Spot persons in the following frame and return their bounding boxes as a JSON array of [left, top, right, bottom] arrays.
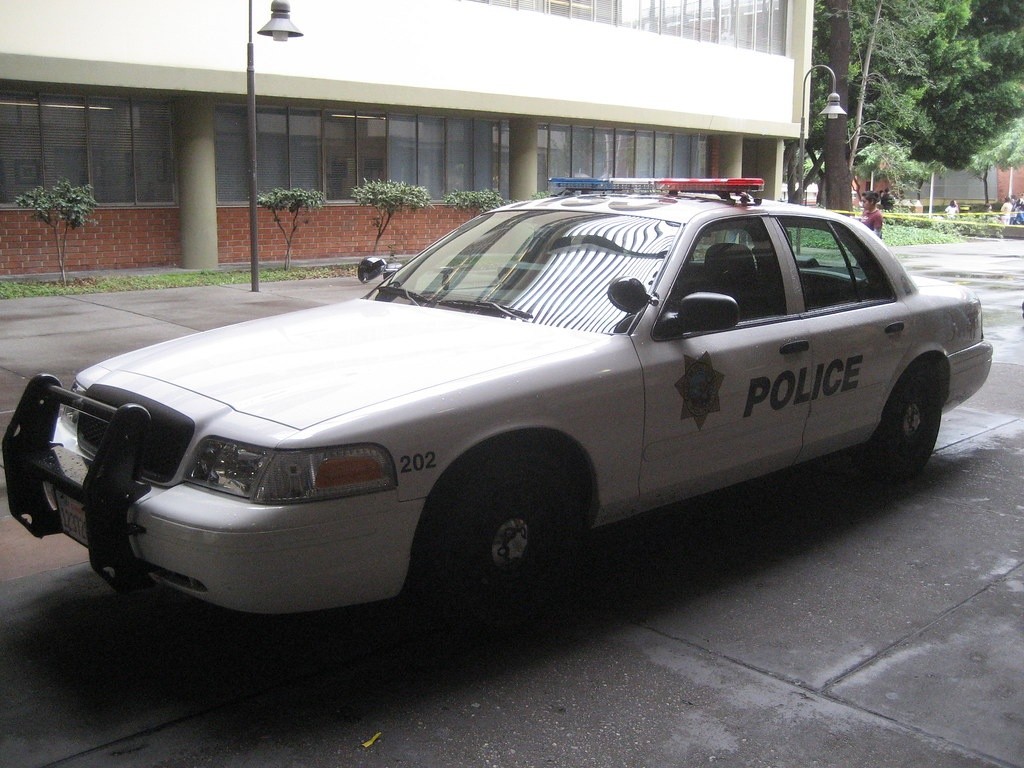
[[944, 200, 959, 218], [859, 191, 883, 240], [881, 187, 895, 209], [1001, 193, 1024, 225]]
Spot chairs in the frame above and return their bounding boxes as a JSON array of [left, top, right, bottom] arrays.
[[704, 242, 772, 323]]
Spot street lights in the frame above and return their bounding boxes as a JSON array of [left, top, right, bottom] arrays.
[[797, 66, 847, 257], [246, 0, 306, 290]]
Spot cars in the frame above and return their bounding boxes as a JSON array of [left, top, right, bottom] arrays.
[[3, 176, 993, 641]]
